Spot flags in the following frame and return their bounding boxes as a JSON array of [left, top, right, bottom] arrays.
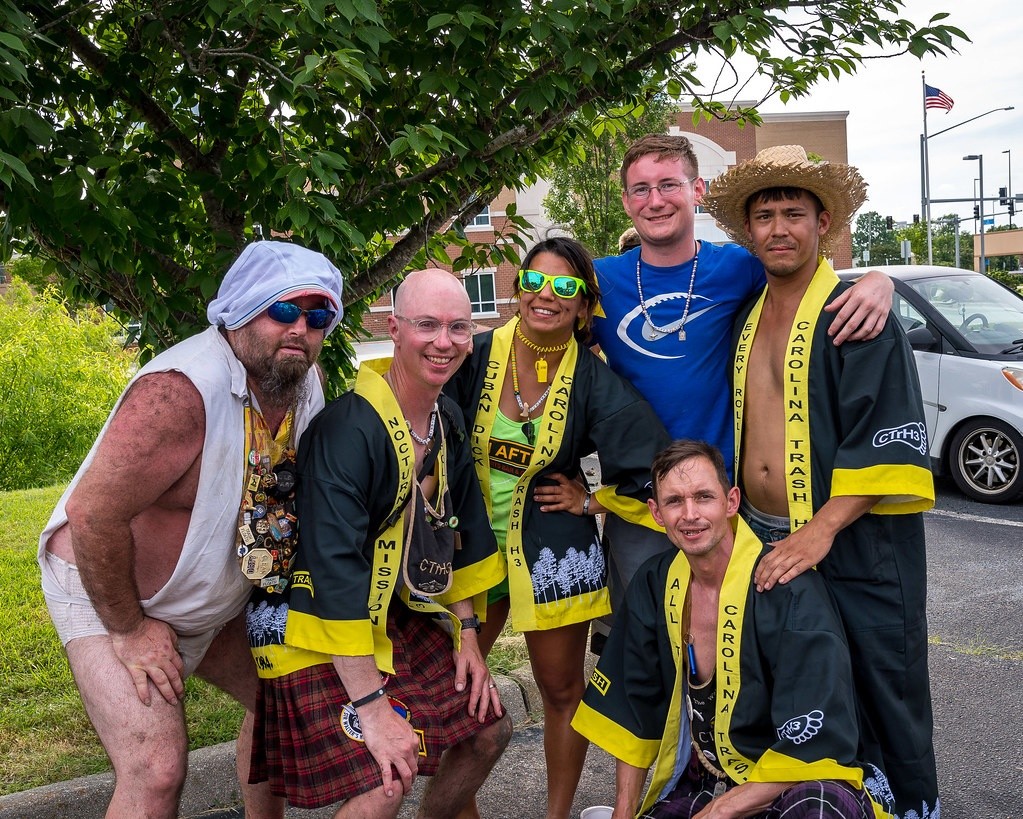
[[926, 84, 955, 114]]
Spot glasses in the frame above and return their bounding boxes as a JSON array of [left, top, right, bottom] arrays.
[[518, 268, 588, 298], [623, 176, 699, 201], [395, 314, 478, 344], [267, 302, 336, 330]]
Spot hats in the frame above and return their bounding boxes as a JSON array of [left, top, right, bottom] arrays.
[[701, 146, 869, 257], [281, 283, 337, 310]]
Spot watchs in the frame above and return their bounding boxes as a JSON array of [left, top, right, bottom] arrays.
[[460, 614, 481, 634]]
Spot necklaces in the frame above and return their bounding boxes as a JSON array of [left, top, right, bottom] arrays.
[[515, 321, 572, 351], [637, 239, 698, 341], [405, 411, 437, 444], [511, 340, 552, 412]]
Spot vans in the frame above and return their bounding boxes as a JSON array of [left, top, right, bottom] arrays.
[[833, 264, 1023, 505]]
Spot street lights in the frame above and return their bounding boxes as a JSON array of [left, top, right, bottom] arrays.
[[962, 154, 986, 275], [920, 106, 1014, 224], [1003, 150, 1012, 229]]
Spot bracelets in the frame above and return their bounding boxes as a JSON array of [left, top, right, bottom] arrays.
[[583, 493, 591, 514], [352, 687, 386, 708]]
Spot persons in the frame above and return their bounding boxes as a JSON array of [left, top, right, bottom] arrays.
[[701, 144, 941, 819], [441, 236, 673, 819], [245, 267, 513, 819], [37, 240, 343, 819], [569, 440, 864, 819], [468, 134, 895, 656]]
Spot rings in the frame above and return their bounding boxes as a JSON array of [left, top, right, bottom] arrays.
[[489, 684, 496, 689]]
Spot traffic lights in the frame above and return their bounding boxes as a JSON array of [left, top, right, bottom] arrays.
[[886, 217, 894, 230], [974, 204, 979, 220], [1007, 201, 1014, 216]]
